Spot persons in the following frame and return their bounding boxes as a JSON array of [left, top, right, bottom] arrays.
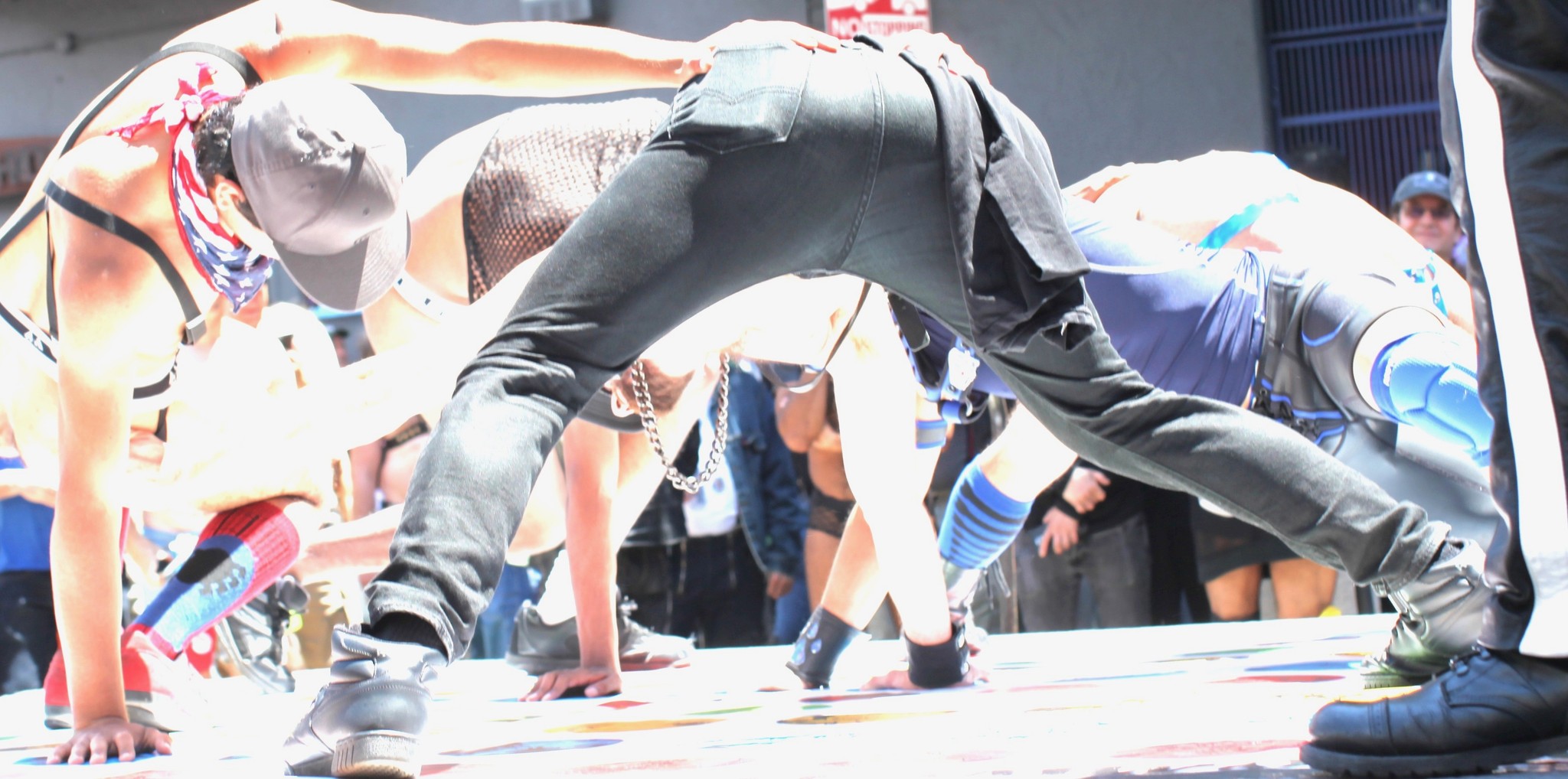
[[0, 0, 1568, 779]]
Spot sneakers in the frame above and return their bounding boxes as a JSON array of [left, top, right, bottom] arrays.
[[42, 648, 75, 728], [120, 625, 195, 732]]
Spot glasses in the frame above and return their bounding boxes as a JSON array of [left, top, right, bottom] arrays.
[[1406, 207, 1454, 219]]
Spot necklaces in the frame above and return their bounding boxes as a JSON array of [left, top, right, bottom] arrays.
[[631, 344, 730, 493]]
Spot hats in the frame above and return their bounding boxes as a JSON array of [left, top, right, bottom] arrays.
[[1391, 171, 1462, 217], [231, 74, 410, 312]]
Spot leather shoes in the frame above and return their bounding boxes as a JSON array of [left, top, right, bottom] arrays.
[[215, 574, 307, 696], [1343, 538, 1488, 694], [1299, 648, 1568, 778], [505, 596, 694, 674], [278, 626, 445, 777]]
